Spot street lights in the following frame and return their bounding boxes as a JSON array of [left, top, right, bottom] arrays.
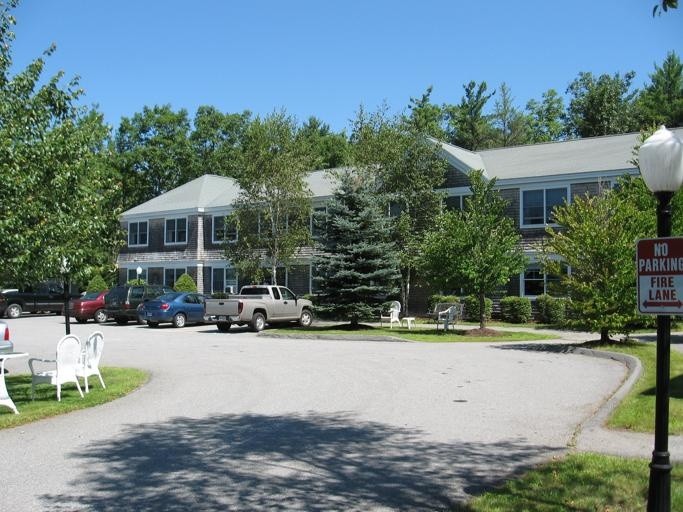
[[637, 126, 683, 512]]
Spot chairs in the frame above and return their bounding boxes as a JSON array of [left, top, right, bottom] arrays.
[[26, 330, 105, 402], [380, 299, 402, 329], [436, 306, 457, 331]]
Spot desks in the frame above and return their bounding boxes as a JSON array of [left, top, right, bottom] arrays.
[[401, 317, 416, 330], [0, 351, 29, 415]]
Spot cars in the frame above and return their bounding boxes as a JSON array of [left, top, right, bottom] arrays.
[[136, 290, 208, 328], [0, 320, 15, 361], [69, 288, 111, 325]]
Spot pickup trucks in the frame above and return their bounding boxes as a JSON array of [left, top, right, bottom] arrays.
[[0, 282, 82, 319], [204, 284, 315, 332]]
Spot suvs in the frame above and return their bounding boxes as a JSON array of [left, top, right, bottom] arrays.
[[104, 285, 173, 325]]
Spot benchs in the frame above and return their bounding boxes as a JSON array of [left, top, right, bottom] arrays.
[[427, 302, 464, 324]]
